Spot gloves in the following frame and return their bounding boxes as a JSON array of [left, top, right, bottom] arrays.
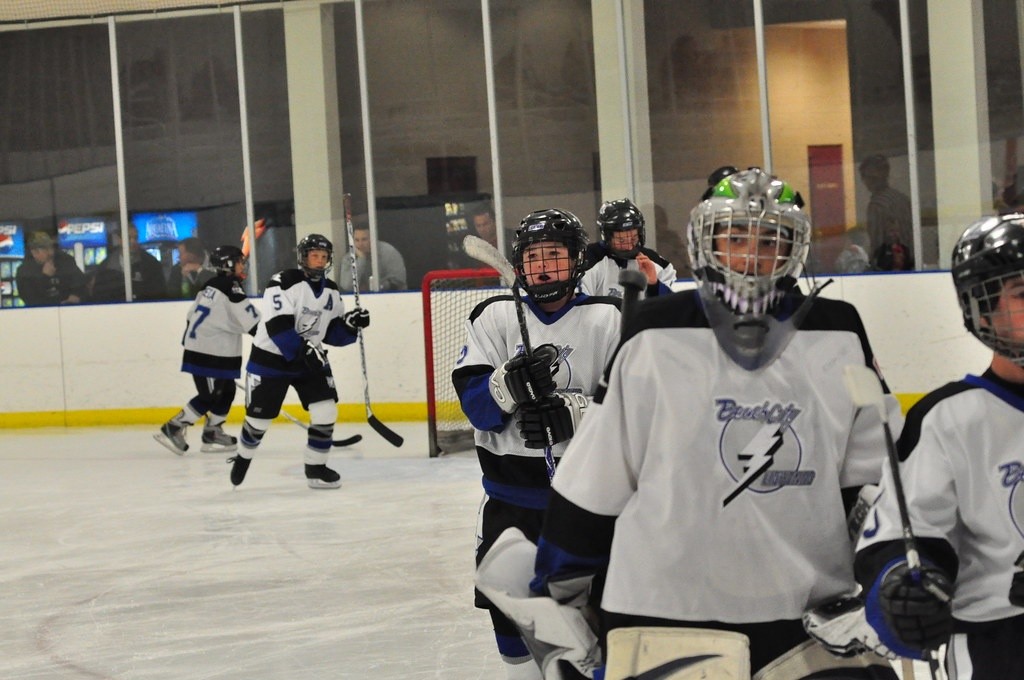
[[345, 308, 369, 328], [490, 353, 557, 415], [304, 340, 325, 374], [882, 564, 952, 650], [515, 393, 589, 449]]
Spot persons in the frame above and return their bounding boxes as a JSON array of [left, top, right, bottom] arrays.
[[91, 224, 165, 303], [164, 236, 216, 300], [16, 231, 89, 307], [528, 171, 902, 680], [339, 223, 406, 292], [450, 209, 625, 680], [226, 234, 371, 485], [852, 214, 1024, 680], [447, 207, 518, 290], [160, 245, 262, 451], [573, 199, 677, 303], [844, 152, 913, 276], [868, 220, 915, 271], [654, 204, 694, 278]]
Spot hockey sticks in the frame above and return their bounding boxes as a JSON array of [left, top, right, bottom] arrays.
[[343, 191, 405, 449], [232, 376, 363, 447], [845, 363, 951, 680], [462, 234, 558, 487]]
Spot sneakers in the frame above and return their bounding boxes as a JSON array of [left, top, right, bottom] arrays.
[[153, 409, 194, 456], [227, 454, 252, 489], [200, 413, 237, 452], [305, 463, 341, 488]]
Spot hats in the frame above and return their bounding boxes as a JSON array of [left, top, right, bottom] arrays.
[[27, 231, 58, 248]]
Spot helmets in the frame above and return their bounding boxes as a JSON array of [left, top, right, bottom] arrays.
[[296, 234, 335, 281], [596, 198, 646, 260], [951, 213, 1024, 369], [210, 245, 248, 280], [511, 208, 590, 303], [686, 168, 810, 315]]
